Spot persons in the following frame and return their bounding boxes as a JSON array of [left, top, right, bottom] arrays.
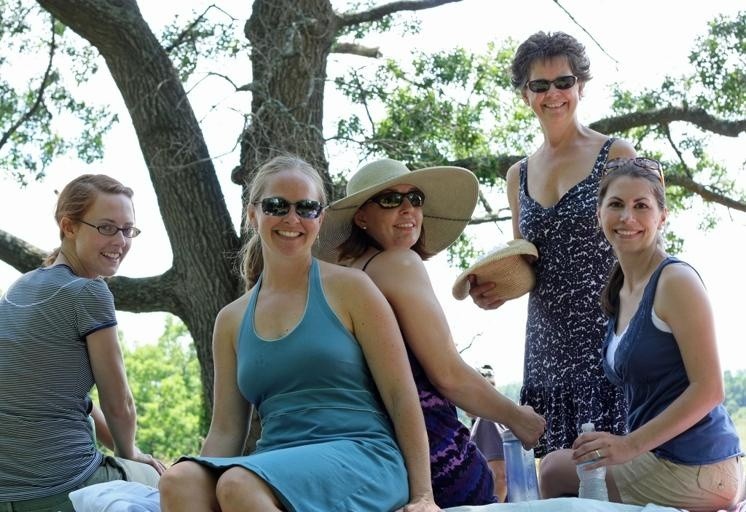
[[462, 363, 508, 504], [0, 172, 171, 512], [462, 30, 640, 499], [156, 153, 444, 510], [334, 182, 549, 508], [530, 152, 744, 511]]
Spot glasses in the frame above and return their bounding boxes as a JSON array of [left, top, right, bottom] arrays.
[[70, 217, 142, 239], [249, 197, 324, 221], [599, 156, 666, 199], [526, 73, 579, 94], [361, 187, 426, 209]]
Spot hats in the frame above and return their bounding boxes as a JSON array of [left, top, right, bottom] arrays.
[[451, 238, 541, 304], [310, 158, 479, 268]]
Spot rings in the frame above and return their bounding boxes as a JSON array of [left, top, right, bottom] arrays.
[[592, 448, 602, 458]]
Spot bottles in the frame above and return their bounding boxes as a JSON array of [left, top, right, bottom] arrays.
[[575, 423, 610, 498], [501, 428, 540, 502]]
